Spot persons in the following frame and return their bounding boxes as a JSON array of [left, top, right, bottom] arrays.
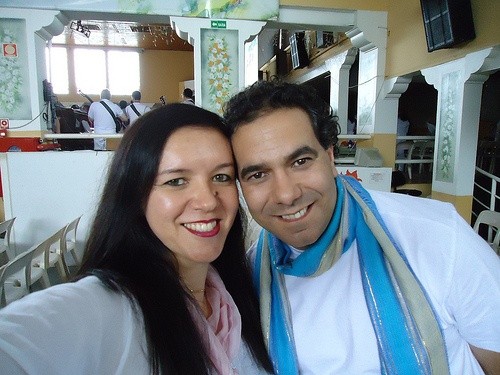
[[47, 84, 195, 151], [0, 105, 278, 375], [223, 77, 500, 375]]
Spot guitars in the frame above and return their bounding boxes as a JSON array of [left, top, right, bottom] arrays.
[[78, 90, 93, 104], [159, 95, 169, 105]]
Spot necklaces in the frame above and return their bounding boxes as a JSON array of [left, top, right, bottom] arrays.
[[183, 281, 207, 297]]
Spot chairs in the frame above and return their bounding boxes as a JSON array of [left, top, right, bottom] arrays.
[[472, 210, 500, 254], [397, 140, 433, 179], [0, 216, 82, 307]]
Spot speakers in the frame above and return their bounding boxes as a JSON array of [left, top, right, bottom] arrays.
[[419, 0, 476, 52], [289, 32, 309, 70]]
